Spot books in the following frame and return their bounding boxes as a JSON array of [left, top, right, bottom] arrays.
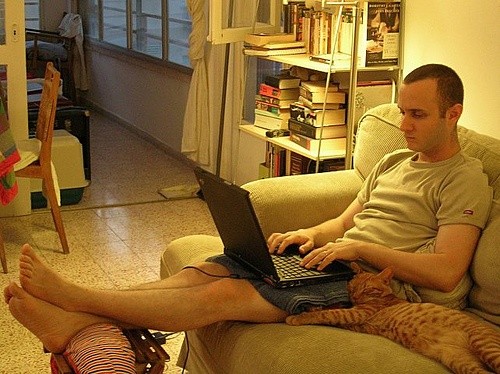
[[254, 76, 300, 130], [287, 79, 348, 150], [242, 1, 399, 65], [257, 142, 346, 180]]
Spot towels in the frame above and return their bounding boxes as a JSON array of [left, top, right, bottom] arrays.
[[59, 13, 88, 91]]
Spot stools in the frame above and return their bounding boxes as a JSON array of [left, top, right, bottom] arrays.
[[43, 325, 170, 374]]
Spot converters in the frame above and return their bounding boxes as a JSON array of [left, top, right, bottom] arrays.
[[152, 332, 166, 344]]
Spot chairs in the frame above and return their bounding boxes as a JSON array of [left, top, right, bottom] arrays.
[[0, 62, 69, 274], [25, 11, 73, 83]]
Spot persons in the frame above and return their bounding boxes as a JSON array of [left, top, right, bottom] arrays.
[[3, 64, 495, 355]]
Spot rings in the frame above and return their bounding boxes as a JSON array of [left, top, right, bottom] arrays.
[[323, 251, 328, 255]]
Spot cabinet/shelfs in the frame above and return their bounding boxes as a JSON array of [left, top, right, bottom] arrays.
[[233, 0, 405, 187]]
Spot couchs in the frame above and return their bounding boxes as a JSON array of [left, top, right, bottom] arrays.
[[160, 103, 500, 374]]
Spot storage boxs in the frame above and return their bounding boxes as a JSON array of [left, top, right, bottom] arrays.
[[28, 129, 89, 209]]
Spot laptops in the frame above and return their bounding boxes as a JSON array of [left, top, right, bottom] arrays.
[[194, 166, 354, 289]]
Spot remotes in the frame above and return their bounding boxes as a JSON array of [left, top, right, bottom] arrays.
[[266, 129, 290, 138]]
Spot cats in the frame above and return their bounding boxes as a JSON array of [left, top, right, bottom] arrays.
[[285, 261, 500, 374]]
